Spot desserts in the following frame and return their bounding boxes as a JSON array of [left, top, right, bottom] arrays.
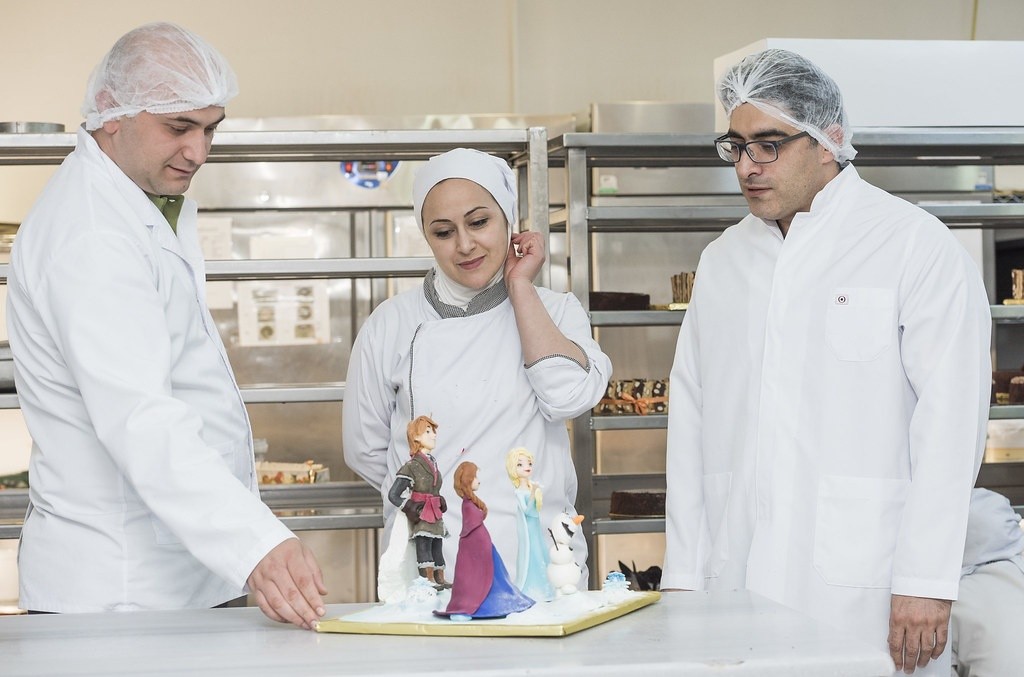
[[590, 291, 651, 311]]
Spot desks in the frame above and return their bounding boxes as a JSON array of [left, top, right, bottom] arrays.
[[0, 590, 899, 677]]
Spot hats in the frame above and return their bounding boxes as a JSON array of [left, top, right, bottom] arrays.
[[413, 148, 516, 237]]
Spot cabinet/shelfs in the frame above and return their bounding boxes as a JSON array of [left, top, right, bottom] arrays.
[[506, 133, 1024, 590], [0, 127, 549, 605]]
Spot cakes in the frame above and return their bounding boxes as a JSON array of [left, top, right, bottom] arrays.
[[610, 488, 667, 516], [592, 378, 670, 415], [670, 271, 696, 303]]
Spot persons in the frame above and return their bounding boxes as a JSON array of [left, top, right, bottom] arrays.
[[10, 25, 330, 636], [661, 52, 993, 677], [341, 149, 614, 605], [950, 488, 1021, 676], [389, 416, 549, 623]]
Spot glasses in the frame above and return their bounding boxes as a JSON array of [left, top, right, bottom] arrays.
[[714, 128, 808, 164]]
